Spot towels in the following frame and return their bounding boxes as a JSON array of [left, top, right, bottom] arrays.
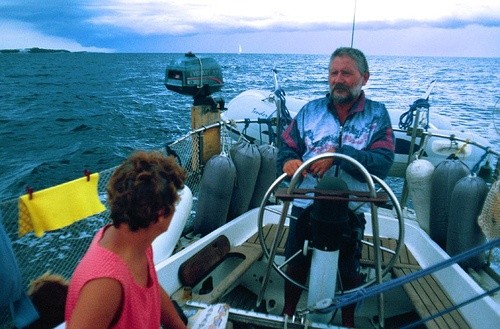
[[18, 172, 108, 238]]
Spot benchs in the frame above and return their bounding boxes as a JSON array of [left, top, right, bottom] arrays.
[[393, 268, 471, 329], [242, 223, 421, 269]]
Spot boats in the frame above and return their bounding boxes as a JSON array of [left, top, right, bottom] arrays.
[[219, 90, 491, 163]]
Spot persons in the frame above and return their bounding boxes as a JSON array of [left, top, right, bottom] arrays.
[[277, 47, 396, 329], [65, 152, 186, 329]]
